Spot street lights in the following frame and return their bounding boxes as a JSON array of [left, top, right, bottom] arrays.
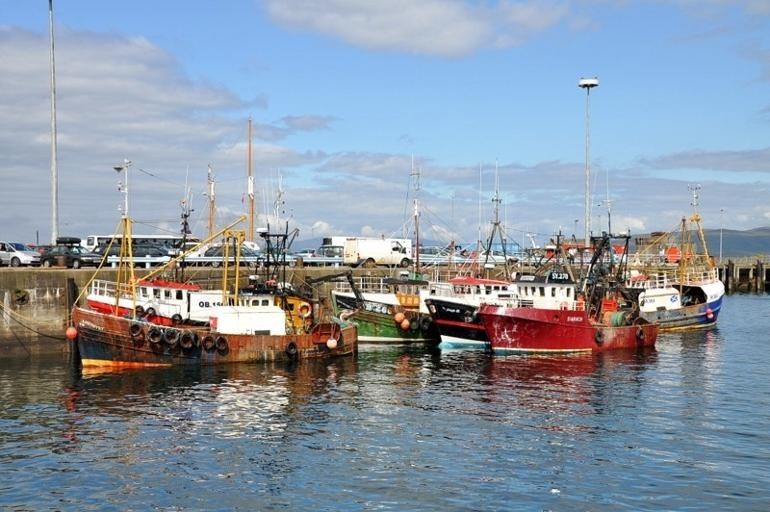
[[578, 76, 601, 251]]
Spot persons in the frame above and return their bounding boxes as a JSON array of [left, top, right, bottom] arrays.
[[634, 249, 642, 267], [567, 245, 578, 264]]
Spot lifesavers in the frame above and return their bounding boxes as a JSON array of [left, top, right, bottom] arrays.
[[297, 302, 312, 318]]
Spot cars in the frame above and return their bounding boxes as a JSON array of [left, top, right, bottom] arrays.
[[0, 241, 42, 267], [41, 237, 106, 270]]
